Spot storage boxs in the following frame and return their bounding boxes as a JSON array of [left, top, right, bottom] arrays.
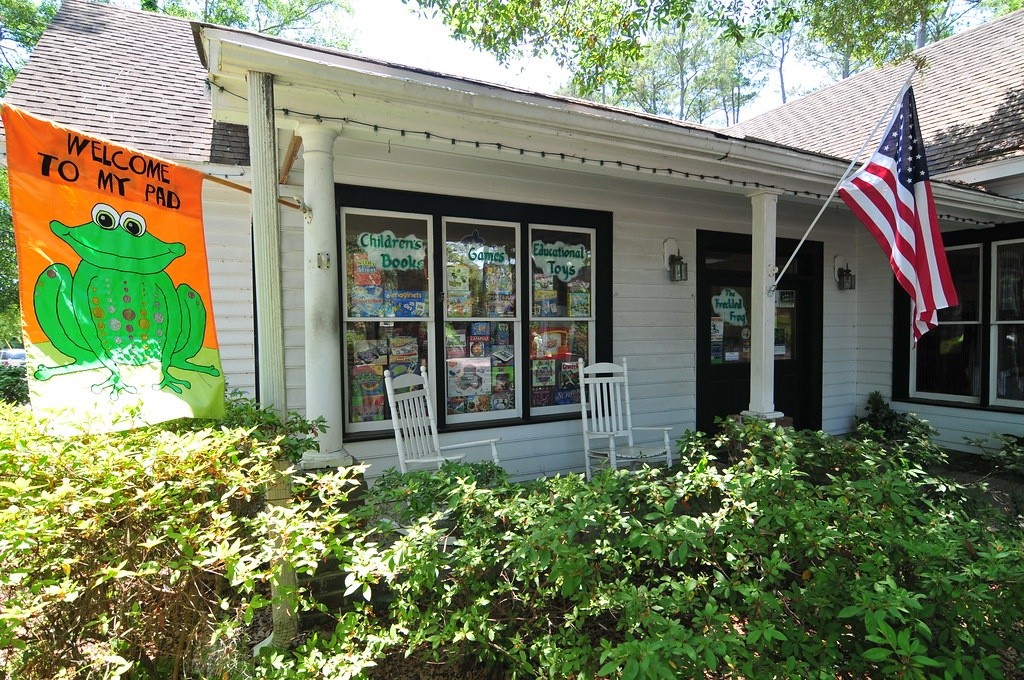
[[347, 254, 590, 423]]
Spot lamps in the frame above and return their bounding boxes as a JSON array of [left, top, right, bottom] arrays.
[[664, 236, 689, 284], [834, 253, 856, 291]]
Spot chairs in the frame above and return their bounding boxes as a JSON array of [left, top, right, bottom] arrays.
[[578, 357, 674, 481], [384, 365, 502, 472]]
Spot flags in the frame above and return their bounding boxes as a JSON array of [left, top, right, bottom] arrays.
[[837, 79, 959, 351]]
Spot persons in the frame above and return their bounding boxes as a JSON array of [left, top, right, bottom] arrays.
[[916, 291, 977, 397]]
